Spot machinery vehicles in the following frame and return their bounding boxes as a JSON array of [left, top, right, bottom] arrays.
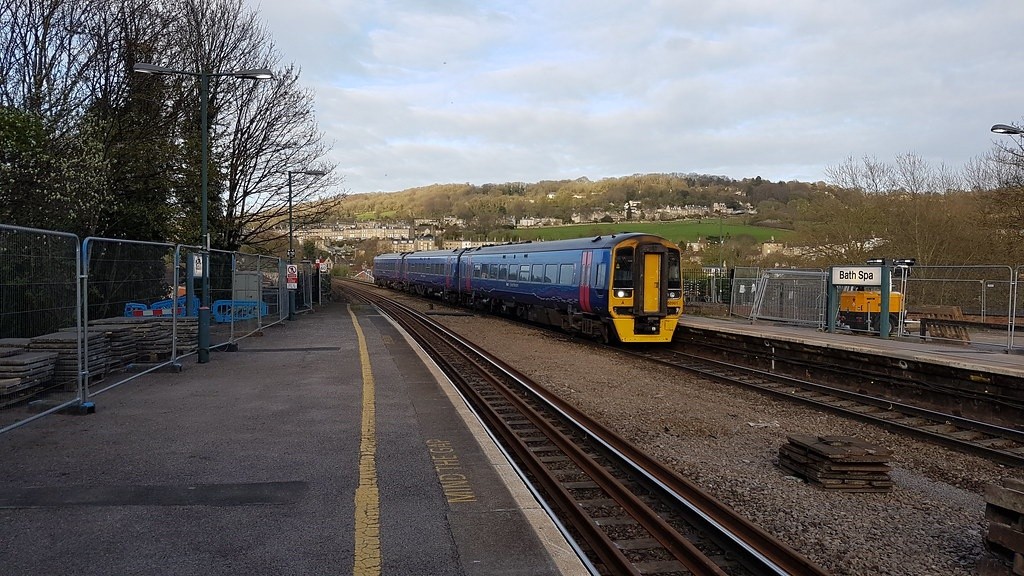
[[840, 260, 921, 337]]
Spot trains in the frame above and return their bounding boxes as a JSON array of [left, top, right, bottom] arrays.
[[372, 231, 684, 346]]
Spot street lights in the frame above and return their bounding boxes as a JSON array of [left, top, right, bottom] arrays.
[[714, 209, 723, 303], [289, 171, 325, 322], [132, 63, 273, 363]]
